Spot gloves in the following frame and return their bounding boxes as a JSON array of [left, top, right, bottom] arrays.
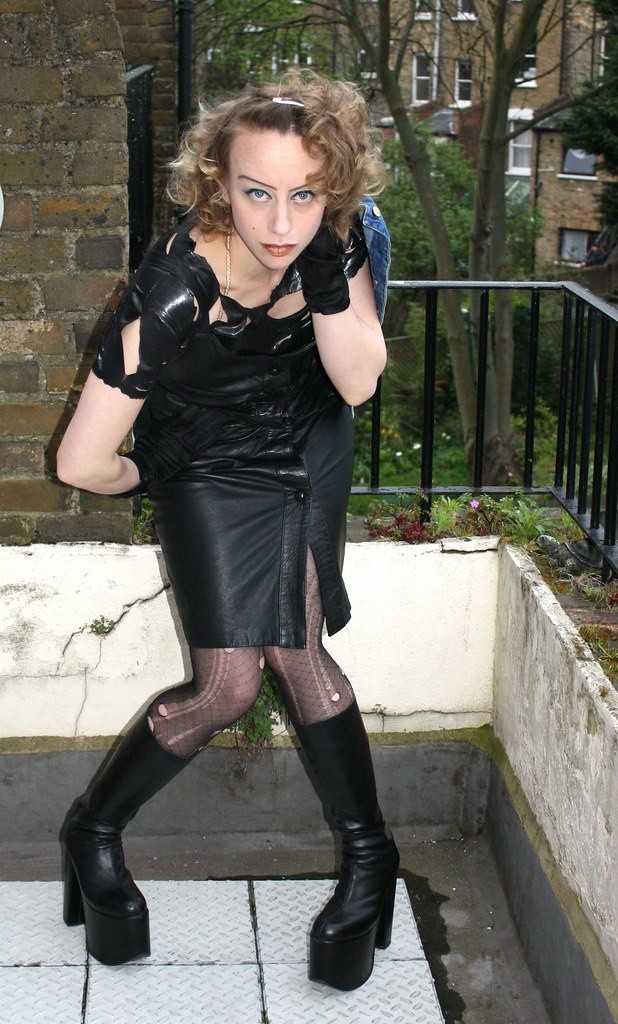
[[295, 218, 352, 316], [110, 404, 225, 499]]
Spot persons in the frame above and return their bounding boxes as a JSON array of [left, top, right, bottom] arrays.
[[57, 66, 401, 991]]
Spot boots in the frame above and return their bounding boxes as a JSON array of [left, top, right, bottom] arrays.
[[286, 671, 397, 991], [59, 707, 203, 966]]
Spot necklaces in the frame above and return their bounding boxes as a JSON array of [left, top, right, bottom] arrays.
[[218, 224, 231, 320]]
